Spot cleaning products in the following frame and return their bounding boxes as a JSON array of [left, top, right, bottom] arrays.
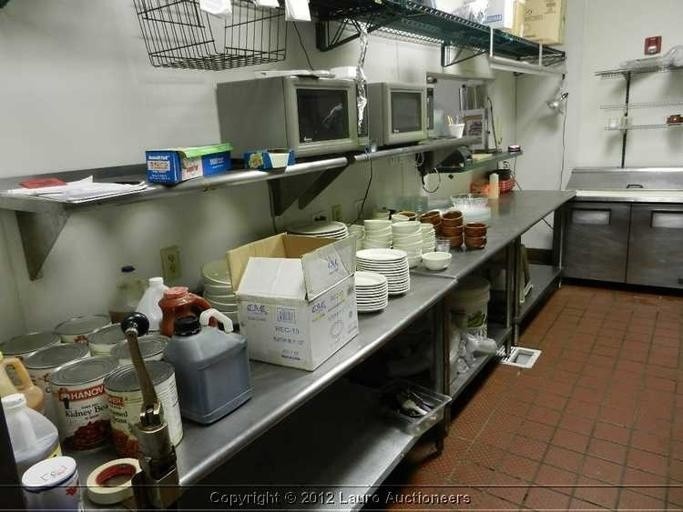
[[490, 171, 499, 199]]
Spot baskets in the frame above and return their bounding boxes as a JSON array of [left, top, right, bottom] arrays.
[[132, 1, 288, 72]]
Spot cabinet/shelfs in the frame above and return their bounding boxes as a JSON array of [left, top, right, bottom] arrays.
[[552, 194, 683, 288], [0, 0, 564, 282], [593, 62, 683, 135]]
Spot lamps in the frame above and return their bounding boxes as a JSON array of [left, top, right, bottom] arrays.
[[542, 86, 569, 115]]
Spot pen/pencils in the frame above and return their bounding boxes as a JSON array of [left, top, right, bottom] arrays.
[[447, 114, 458, 125]]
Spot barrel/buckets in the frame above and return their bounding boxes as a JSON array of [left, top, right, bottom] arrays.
[[2, 392, 62, 477], [136, 277, 166, 330], [165, 308, 253, 425], [0, 352, 45, 415], [449, 278, 491, 342], [161, 289, 219, 333]]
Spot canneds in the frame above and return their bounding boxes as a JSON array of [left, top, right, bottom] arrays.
[[0, 314, 185, 460]]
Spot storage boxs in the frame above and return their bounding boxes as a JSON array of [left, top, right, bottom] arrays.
[[225, 230, 361, 373]]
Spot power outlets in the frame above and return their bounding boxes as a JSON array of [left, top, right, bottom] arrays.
[[160, 246, 181, 281]]
